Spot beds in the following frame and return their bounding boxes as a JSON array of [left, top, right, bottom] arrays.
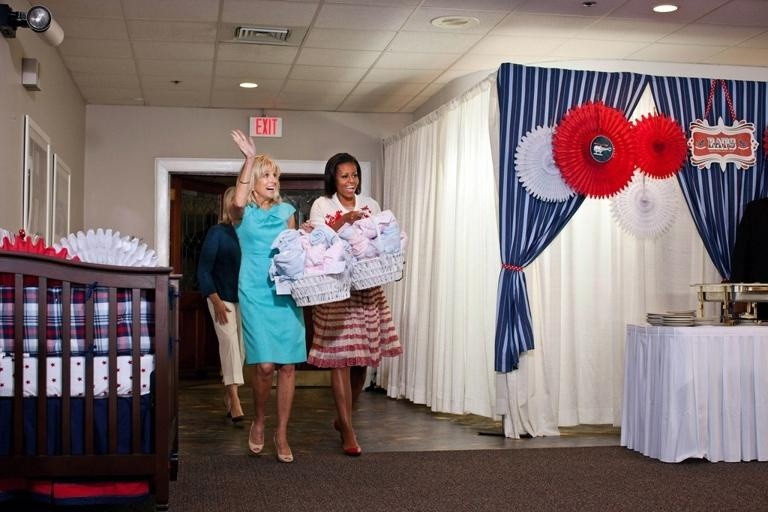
[[0, 222, 190, 511]]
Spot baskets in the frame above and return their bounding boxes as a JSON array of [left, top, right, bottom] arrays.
[[349, 249, 403, 292], [288, 266, 351, 307]]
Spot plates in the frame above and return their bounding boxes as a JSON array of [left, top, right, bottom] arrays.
[[647, 311, 697, 327]]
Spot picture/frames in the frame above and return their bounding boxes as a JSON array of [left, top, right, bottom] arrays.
[[49, 152, 74, 250], [18, 114, 54, 248]]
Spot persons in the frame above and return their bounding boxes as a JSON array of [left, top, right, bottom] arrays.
[[196, 185, 248, 425], [227, 129, 309, 464], [309, 153, 406, 458], [729, 194, 768, 321]]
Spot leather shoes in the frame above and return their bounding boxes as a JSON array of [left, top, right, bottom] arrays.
[[335, 417, 362, 456], [223, 400, 245, 422]]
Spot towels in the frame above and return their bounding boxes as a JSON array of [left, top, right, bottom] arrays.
[[269, 208, 409, 295]]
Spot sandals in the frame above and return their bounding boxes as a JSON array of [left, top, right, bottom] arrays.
[[273, 432, 294, 463], [249, 421, 264, 454]]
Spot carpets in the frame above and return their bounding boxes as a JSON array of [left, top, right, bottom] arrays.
[[161, 436, 766, 511]]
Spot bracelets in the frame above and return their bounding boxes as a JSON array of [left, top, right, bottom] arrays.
[[238, 177, 251, 185]]
